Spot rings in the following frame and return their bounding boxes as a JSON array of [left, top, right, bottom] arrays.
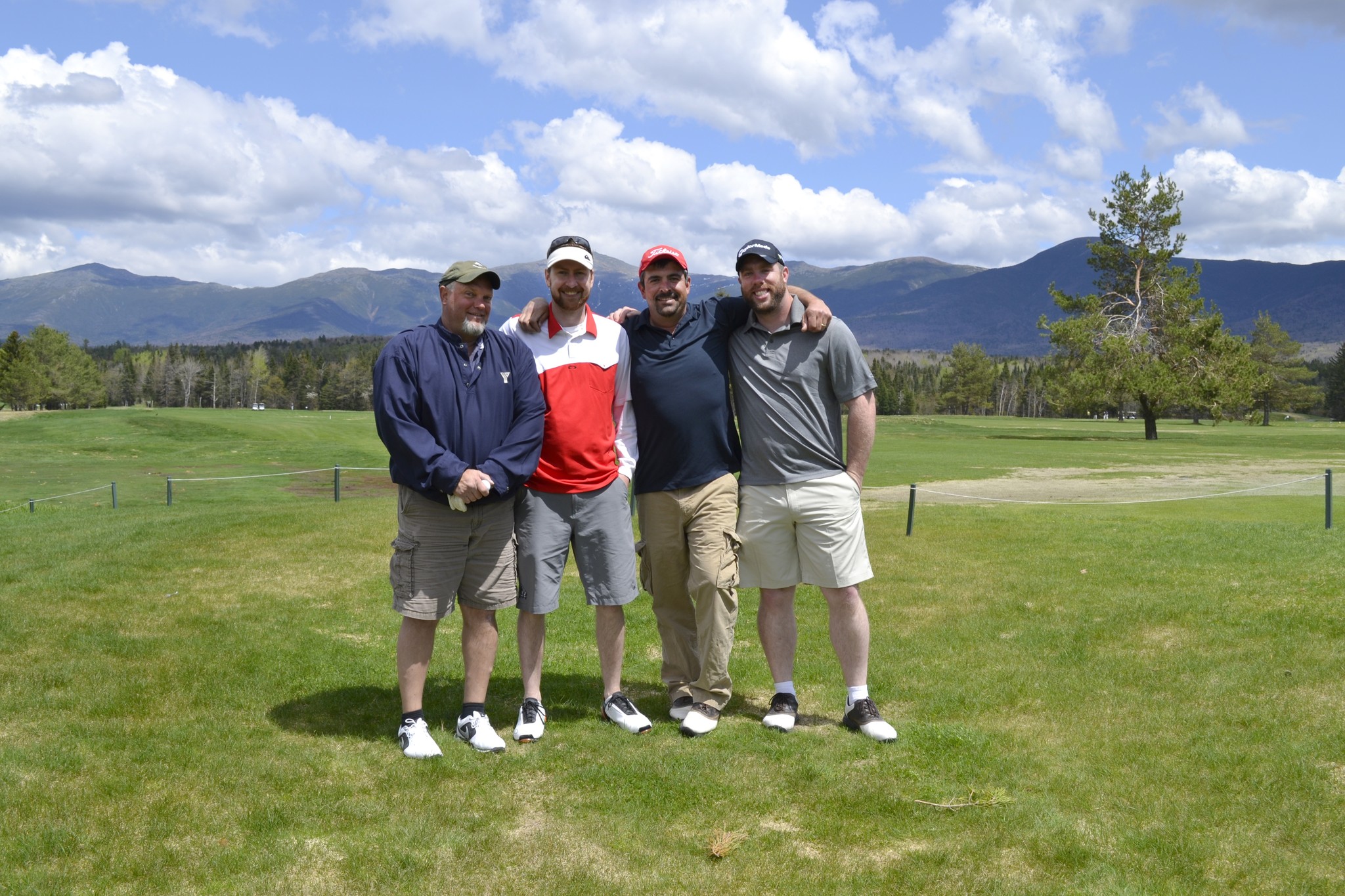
[[822, 323, 827, 327]]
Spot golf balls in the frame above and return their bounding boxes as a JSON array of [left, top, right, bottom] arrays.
[[482, 480, 491, 491]]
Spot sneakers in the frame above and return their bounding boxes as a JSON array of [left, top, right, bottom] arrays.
[[454, 711, 506, 754], [669, 695, 694, 722], [762, 693, 799, 733], [842, 695, 898, 744], [679, 702, 720, 738], [513, 697, 546, 744], [602, 691, 653, 736], [398, 717, 444, 760]]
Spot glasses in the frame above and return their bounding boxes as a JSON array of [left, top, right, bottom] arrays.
[[548, 236, 591, 256]]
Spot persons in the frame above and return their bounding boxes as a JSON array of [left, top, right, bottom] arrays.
[[606, 239, 899, 744], [1103, 412, 1106, 419], [1093, 412, 1097, 419], [519, 244, 834, 738], [290, 403, 294, 410], [1106, 410, 1108, 419], [370, 260, 548, 763], [496, 236, 655, 745]]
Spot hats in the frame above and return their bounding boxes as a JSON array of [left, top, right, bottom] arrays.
[[736, 239, 785, 276], [639, 245, 688, 279], [439, 260, 501, 290], [547, 246, 594, 270]]
